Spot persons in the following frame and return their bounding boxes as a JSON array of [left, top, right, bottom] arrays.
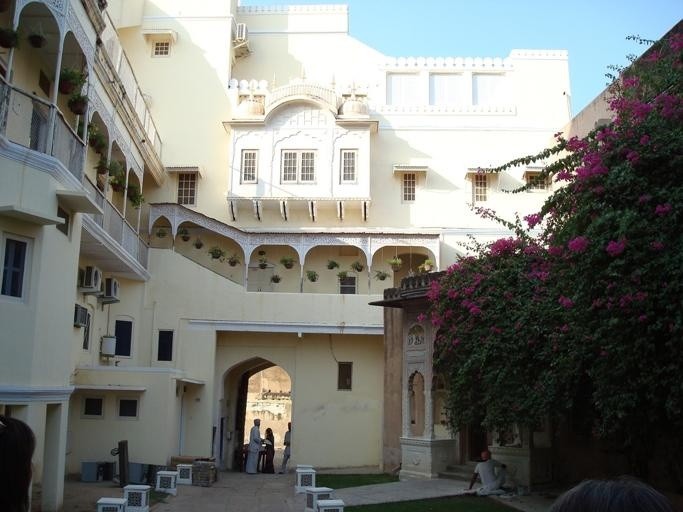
[[277, 421, 291, 474], [264, 428, 275, 474], [245, 418, 265, 474], [461, 449, 517, 498], [548, 469, 672, 512]]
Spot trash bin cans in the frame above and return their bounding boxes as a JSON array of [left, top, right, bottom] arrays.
[[193, 462, 215, 487]]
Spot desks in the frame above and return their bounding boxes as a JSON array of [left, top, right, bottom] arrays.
[[237, 444, 266, 474]]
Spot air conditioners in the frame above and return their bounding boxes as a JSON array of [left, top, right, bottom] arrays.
[[84, 264, 104, 298], [102, 277, 120, 304]]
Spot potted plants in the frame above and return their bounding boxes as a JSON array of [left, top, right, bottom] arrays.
[[13, 23, 146, 213], [152, 222, 432, 283]]
